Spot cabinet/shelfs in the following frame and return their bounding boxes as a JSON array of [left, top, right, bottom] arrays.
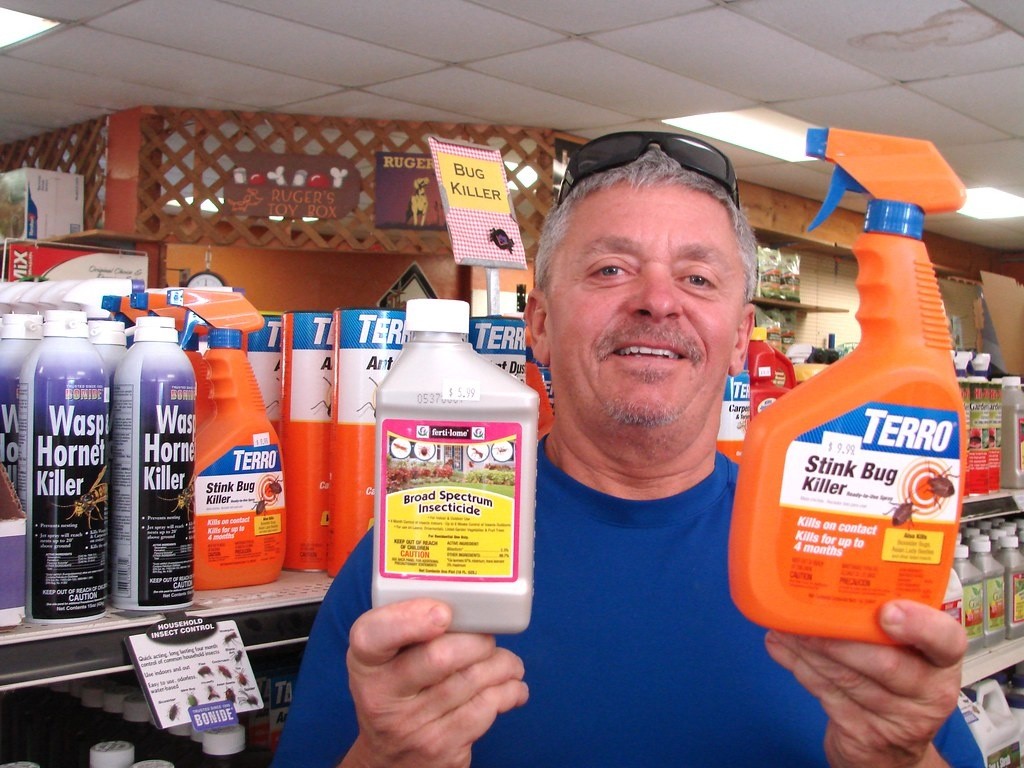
[[0, 488, 1024, 768]]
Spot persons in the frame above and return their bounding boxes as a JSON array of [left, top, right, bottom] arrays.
[[269, 132, 984, 768]]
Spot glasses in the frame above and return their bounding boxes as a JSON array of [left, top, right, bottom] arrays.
[[556, 130, 739, 210]]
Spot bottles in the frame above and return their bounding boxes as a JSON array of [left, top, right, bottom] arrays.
[[111, 316, 196, 610], [0, 312, 45, 631], [371, 299, 541, 635], [0, 674, 253, 768], [936, 517, 1024, 656], [747, 327, 797, 423], [18, 311, 110, 624], [1000, 376, 1024, 489], [957, 664, 1024, 768], [87, 319, 129, 376]]
